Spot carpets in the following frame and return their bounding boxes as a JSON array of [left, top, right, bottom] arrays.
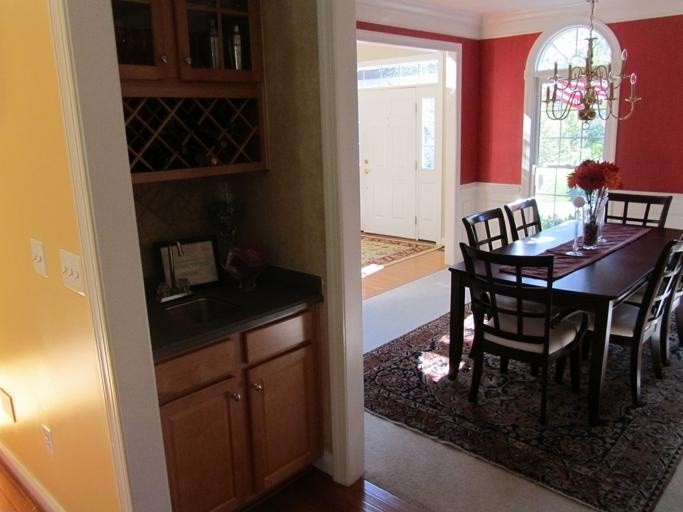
[[361, 233, 443, 280], [362, 299, 683, 512]]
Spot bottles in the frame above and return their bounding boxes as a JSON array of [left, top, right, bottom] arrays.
[[203, 19, 242, 71]]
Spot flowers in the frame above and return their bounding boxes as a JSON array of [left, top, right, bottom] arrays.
[[567, 160, 621, 226]]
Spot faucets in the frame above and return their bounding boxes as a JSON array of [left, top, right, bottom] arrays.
[[168, 240, 188, 291]]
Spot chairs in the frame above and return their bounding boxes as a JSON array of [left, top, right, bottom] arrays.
[[458, 242, 591, 425], [462, 197, 542, 251], [604, 192, 673, 228], [588, 240, 681, 407]]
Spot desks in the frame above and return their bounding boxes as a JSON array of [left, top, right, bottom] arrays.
[[448, 221, 683, 427]]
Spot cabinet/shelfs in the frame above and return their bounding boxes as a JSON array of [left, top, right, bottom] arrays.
[[113, 0, 272, 183], [154, 309, 323, 512]]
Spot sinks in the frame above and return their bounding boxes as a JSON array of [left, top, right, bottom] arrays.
[[162, 296, 241, 323]]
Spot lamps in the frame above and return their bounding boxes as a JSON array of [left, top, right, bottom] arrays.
[[541, 0, 641, 130]]
